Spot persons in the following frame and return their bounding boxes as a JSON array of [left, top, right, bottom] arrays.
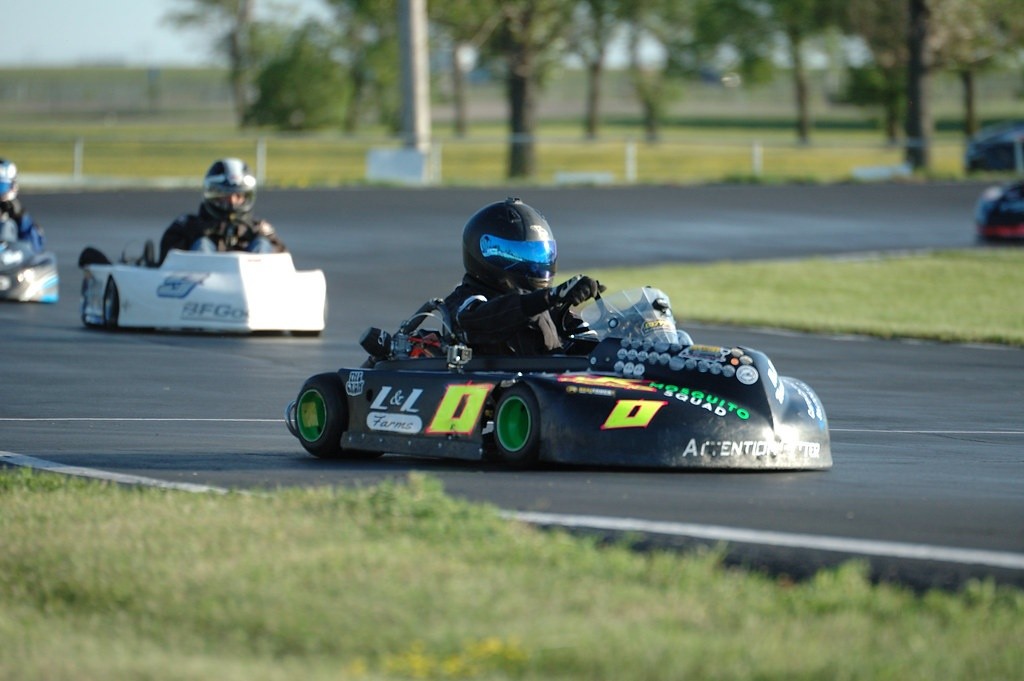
[[444, 197, 606, 356], [0, 159, 44, 243], [160, 158, 287, 263]]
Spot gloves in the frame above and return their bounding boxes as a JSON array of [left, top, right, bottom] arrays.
[[549, 274, 607, 306]]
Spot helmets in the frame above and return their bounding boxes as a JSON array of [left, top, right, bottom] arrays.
[[462, 197, 558, 295], [1, 161, 18, 203], [201, 158, 256, 220]]
